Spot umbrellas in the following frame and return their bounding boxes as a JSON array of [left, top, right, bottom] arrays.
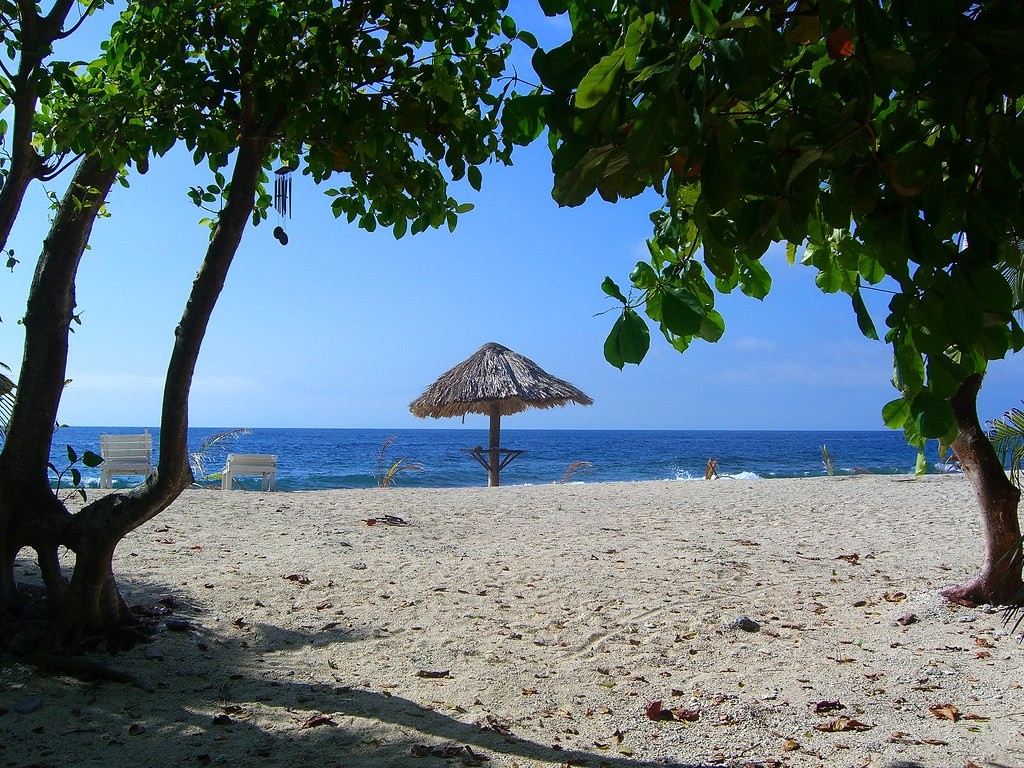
[[408, 343, 594, 487]]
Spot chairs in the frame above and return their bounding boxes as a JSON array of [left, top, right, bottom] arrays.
[[220, 455, 280, 492], [98, 427, 153, 490]]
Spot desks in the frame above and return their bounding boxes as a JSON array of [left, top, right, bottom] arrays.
[[461, 449, 529, 487]]
